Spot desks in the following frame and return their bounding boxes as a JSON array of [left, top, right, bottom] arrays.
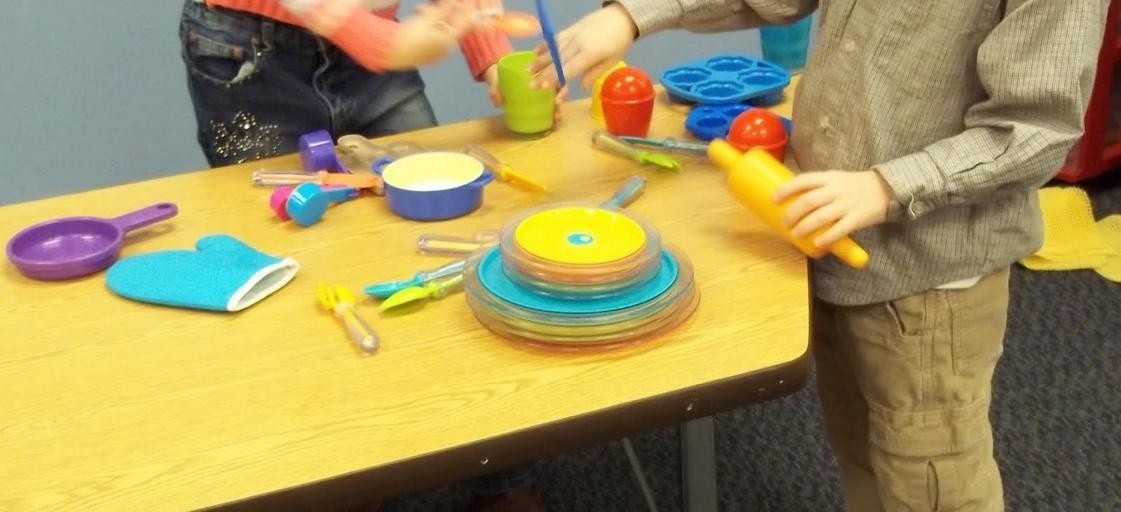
[[0, 65, 813, 512]]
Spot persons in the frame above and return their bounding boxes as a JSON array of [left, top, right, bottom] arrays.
[[178, 0, 564, 169], [529, 0, 1115, 512]]
